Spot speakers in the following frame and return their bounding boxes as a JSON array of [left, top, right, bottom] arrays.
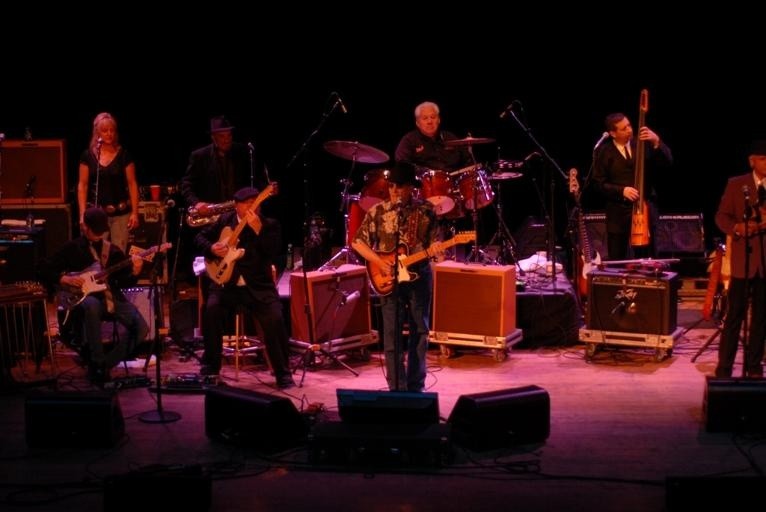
[[448, 385, 551, 458], [24, 390, 125, 452], [204, 383, 302, 450], [704, 376, 765, 426], [337, 390, 439, 463]]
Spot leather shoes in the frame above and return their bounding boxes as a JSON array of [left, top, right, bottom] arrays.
[[200, 364, 219, 376], [276, 374, 294, 388]]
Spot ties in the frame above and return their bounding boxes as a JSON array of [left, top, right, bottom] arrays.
[[624, 146, 630, 159], [758, 185, 766, 206]]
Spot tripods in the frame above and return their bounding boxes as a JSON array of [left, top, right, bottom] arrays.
[[317, 213, 363, 272], [485, 185, 525, 276]]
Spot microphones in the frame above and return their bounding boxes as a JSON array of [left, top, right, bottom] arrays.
[[396, 196, 405, 218], [160, 192, 182, 215], [26, 174, 37, 188], [332, 90, 347, 113], [95, 136, 104, 150], [500, 103, 514, 119], [340, 291, 360, 310], [247, 141, 255, 152], [742, 185, 751, 209]]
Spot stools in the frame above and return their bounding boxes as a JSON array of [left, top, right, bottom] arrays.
[[57, 310, 129, 376], [219, 273, 275, 380]]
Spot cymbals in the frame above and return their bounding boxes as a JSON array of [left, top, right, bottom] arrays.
[[447, 137, 494, 145], [324, 141, 389, 163], [492, 172, 522, 179]]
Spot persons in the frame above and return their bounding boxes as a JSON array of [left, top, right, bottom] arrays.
[[714, 147, 766, 381], [180, 115, 266, 232], [43, 209, 149, 385], [193, 188, 292, 386], [351, 163, 445, 391], [583, 113, 674, 269], [76, 112, 139, 253], [394, 101, 466, 186]]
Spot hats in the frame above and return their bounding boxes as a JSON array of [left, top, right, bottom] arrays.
[[211, 117, 234, 132], [389, 163, 421, 189], [84, 207, 110, 235], [234, 187, 259, 201]]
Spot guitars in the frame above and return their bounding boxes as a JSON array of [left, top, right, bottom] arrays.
[[54, 242, 173, 310], [204, 181, 278, 285], [365, 231, 477, 296], [566, 168, 601, 296]]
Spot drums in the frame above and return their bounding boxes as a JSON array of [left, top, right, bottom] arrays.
[[421, 170, 457, 215], [358, 169, 391, 211], [447, 163, 495, 210]]
